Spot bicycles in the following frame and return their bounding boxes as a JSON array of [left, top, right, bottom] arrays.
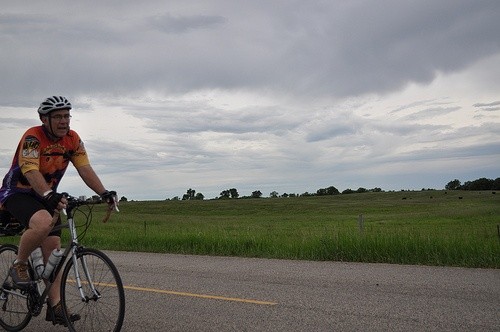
[[0, 191, 125, 332]]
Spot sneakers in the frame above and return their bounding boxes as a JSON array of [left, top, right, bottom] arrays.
[[9, 259, 33, 289], [45, 299, 81, 322]]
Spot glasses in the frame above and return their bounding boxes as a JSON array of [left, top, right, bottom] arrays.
[[46, 113, 70, 120]]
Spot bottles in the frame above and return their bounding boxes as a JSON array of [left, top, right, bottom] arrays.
[[31, 248, 44, 275], [44, 248, 63, 277]]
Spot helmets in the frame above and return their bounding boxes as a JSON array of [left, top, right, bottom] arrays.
[[37, 95, 72, 114]]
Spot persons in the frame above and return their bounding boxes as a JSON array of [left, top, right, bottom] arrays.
[[0, 95, 119, 324]]
